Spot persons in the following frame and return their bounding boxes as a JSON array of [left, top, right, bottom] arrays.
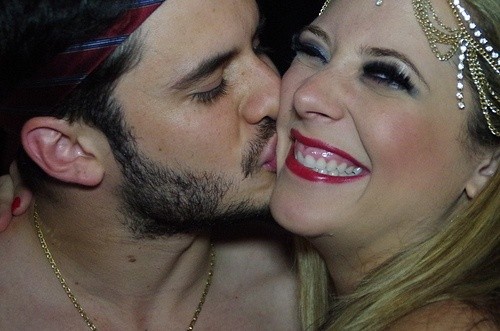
[[0, 0, 304, 330], [1, 0, 500, 330]]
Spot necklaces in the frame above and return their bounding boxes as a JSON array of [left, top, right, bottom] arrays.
[[32, 192, 216, 330]]
